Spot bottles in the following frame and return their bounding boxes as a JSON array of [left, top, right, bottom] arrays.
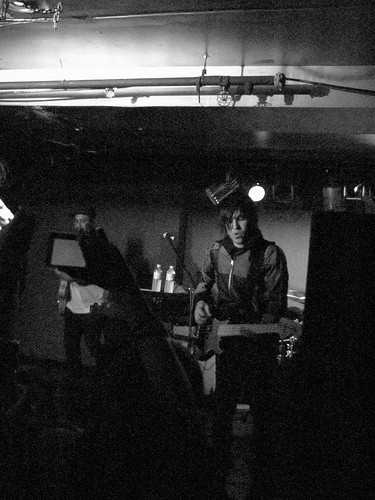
[[164, 266, 177, 293], [152, 264, 163, 292]]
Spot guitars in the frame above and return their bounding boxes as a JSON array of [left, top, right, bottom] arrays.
[[170, 313, 304, 360]]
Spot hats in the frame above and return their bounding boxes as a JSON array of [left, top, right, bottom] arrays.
[[69, 208, 95, 218]]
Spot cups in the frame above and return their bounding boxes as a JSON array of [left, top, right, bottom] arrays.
[[322, 187, 340, 211], [346, 196, 361, 214]]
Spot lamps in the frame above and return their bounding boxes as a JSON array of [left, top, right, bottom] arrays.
[[206, 171, 240, 206]]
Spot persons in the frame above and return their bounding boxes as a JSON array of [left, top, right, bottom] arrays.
[[111, 336, 229, 500], [56, 202, 110, 381], [186, 192, 288, 473]]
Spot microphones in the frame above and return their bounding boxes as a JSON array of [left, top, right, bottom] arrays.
[[162, 232, 175, 241]]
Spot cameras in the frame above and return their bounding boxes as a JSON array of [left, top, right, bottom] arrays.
[[44, 232, 97, 271]]
